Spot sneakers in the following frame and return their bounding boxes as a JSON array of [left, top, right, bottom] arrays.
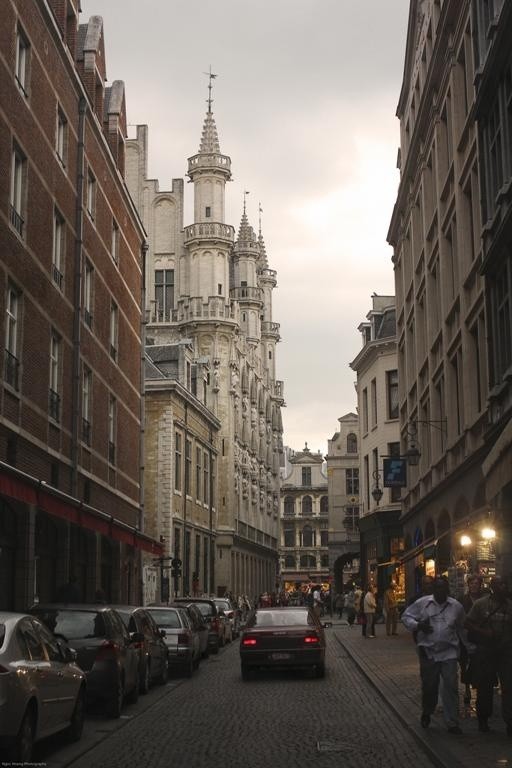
[[421, 690, 512, 736]]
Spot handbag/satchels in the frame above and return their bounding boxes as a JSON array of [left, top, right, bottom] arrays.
[[357, 616, 367, 624]]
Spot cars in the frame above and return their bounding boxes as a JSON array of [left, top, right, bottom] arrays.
[[238, 604, 333, 682], [0, 595, 236, 763]]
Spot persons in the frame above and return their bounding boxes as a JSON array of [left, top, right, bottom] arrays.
[[460, 570, 493, 705], [91, 588, 109, 604], [400, 575, 480, 735], [223, 580, 398, 638], [408, 574, 434, 680], [461, 573, 511, 735], [184, 590, 217, 599]]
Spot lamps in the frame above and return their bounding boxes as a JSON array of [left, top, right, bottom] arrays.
[[371, 416, 447, 505], [460, 510, 495, 547]]
